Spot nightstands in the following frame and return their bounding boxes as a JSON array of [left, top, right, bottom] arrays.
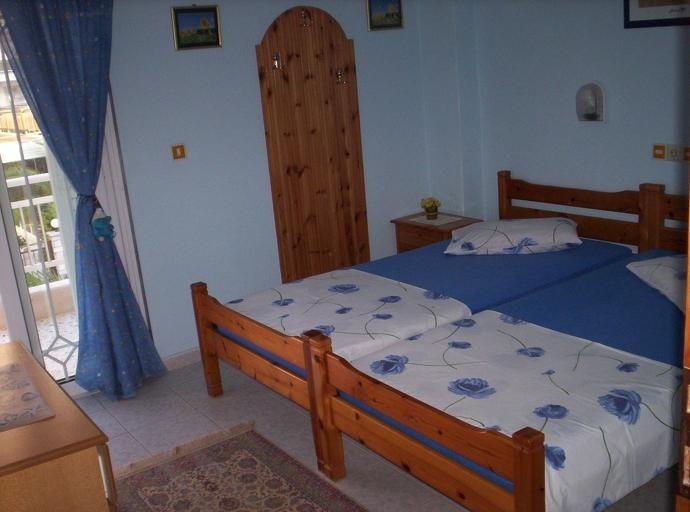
[[389, 212, 483, 254]]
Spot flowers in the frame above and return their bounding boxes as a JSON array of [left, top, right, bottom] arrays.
[[419, 198, 441, 208]]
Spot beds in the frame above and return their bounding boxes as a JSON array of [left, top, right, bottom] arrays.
[[309, 182, 690, 510], [190, 171, 651, 471]]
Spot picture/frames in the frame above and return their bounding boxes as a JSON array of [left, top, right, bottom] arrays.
[[621, 0, 689, 30], [365, 1, 404, 32], [170, 3, 223, 51]]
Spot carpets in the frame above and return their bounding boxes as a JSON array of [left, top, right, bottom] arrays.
[[111, 422, 366, 512]]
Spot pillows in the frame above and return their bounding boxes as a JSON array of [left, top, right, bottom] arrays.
[[443, 216, 585, 255], [626, 252, 687, 314]]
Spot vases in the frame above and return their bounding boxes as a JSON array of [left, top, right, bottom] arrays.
[[426, 207, 436, 216]]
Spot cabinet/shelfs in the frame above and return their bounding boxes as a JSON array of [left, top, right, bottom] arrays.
[[0, 332, 123, 512]]
